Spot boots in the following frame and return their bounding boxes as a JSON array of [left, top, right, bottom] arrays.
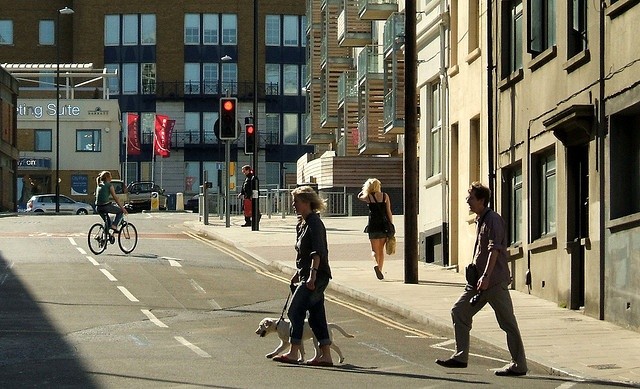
[[242, 217, 252, 227]]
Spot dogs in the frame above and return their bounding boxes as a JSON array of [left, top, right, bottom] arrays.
[[254, 317, 355, 364]]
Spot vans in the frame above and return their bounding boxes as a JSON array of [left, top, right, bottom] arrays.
[[109, 179, 128, 207]]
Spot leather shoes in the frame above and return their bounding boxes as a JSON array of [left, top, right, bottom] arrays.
[[110, 227, 119, 233], [435, 356, 468, 368], [495, 364, 528, 375]]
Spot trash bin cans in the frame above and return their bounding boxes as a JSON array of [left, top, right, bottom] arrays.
[[167, 193, 177, 210]]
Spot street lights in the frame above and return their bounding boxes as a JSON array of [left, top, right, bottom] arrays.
[[55, 4, 75, 213], [218, 53, 232, 94]]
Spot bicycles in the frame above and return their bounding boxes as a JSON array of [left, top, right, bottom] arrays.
[[88, 203, 137, 254]]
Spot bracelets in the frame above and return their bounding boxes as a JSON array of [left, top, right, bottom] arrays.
[[483, 272, 490, 278]]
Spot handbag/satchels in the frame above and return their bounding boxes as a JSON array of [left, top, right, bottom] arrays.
[[466, 261, 488, 290], [385, 235, 397, 256]]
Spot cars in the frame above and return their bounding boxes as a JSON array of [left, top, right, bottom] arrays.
[[27, 193, 94, 214], [187, 193, 236, 212]]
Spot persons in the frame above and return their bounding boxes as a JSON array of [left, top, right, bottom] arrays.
[[309, 267, 318, 272], [95, 171, 128, 246], [436, 183, 528, 377], [238, 165, 261, 227], [357, 179, 395, 279], [272, 186, 334, 367]]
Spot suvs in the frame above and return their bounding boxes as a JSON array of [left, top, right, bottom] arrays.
[[125, 181, 170, 213]]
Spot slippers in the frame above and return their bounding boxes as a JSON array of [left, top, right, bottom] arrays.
[[273, 352, 298, 363], [306, 361, 334, 368]]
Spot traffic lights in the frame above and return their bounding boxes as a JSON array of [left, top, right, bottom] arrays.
[[220, 98, 237, 139], [245, 123, 254, 154]]
